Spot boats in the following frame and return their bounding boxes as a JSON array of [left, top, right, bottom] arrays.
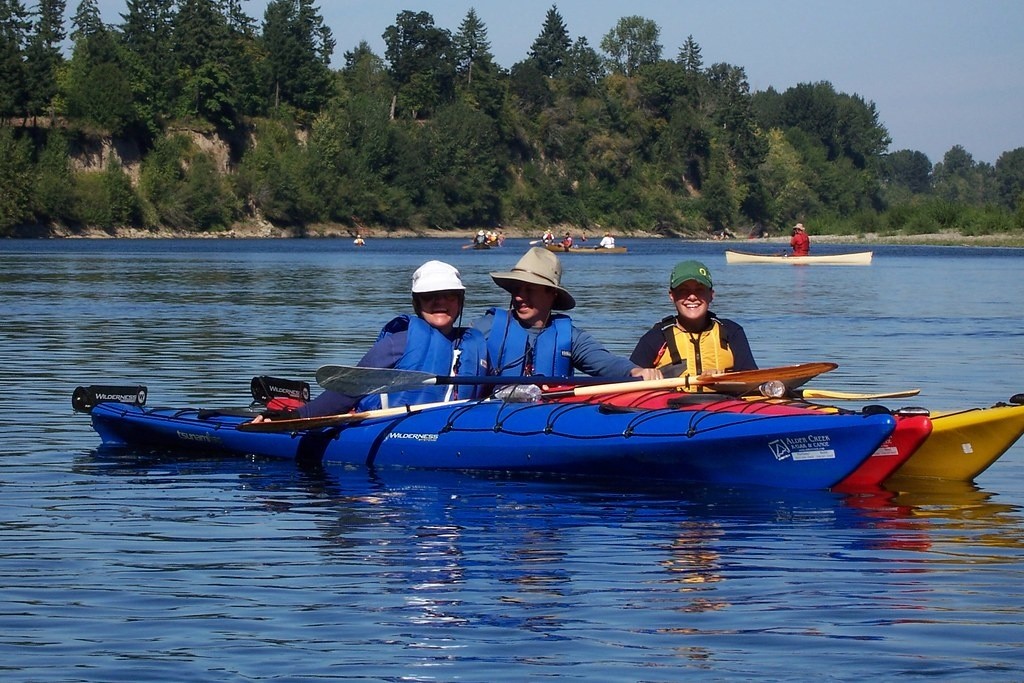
[[546, 243, 629, 253], [724, 248, 873, 265], [72, 374, 1024, 498], [473, 236, 501, 250]]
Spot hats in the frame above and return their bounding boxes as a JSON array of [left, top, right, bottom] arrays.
[[670, 260, 713, 289], [487, 231, 492, 235], [478, 230, 484, 235], [412, 260, 467, 292], [489, 247, 576, 310], [492, 232, 497, 237], [793, 223, 805, 231]]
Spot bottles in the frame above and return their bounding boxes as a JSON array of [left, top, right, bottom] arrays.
[[494, 384, 543, 403], [760, 379, 785, 399]]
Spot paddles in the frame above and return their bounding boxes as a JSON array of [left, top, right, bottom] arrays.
[[678, 388, 924, 400], [512, 361, 839, 399], [235, 397, 473, 432], [315, 365, 649, 397], [462, 242, 477, 249], [529, 240, 543, 244]]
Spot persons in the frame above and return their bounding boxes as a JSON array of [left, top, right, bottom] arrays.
[[630, 260, 758, 393], [558, 232, 572, 247], [582, 232, 586, 241], [251, 260, 496, 423], [599, 232, 615, 249], [353, 235, 365, 245], [472, 246, 663, 399], [790, 222, 810, 257], [474, 230, 505, 243], [542, 229, 554, 247]]
